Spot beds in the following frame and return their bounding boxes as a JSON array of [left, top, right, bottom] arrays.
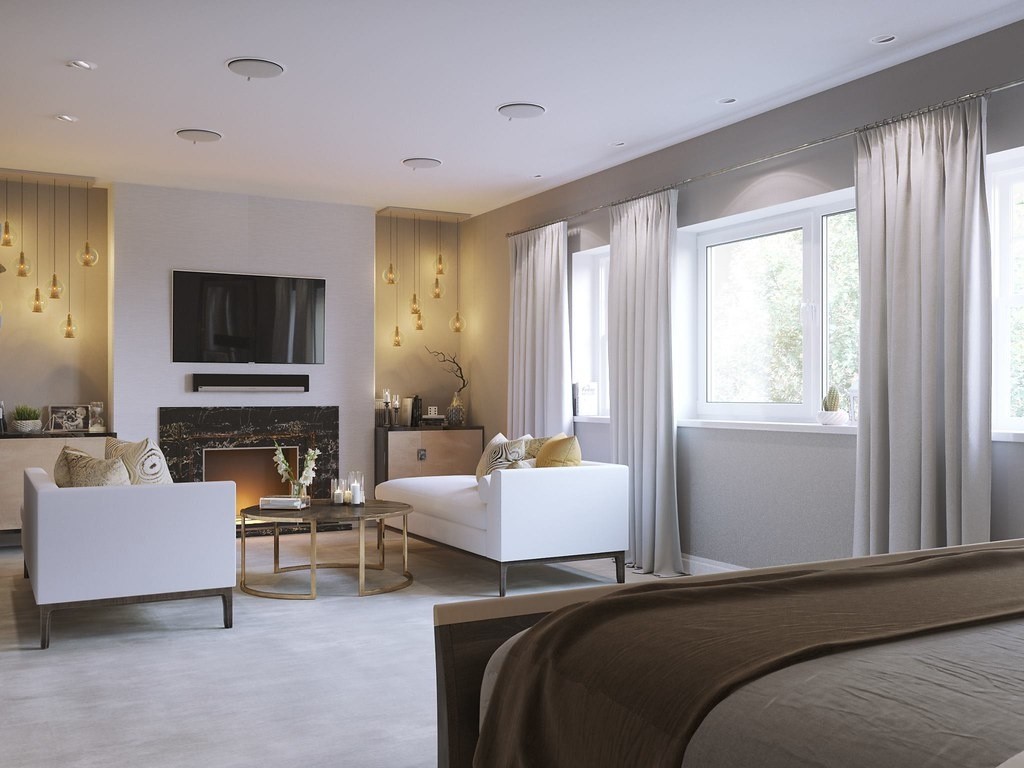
[[435, 538, 1024, 767]]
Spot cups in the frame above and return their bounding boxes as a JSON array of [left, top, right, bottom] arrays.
[[330, 469, 364, 505]]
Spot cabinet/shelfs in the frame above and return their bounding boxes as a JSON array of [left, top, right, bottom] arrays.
[[0, 432, 118, 531], [374, 425, 485, 485]]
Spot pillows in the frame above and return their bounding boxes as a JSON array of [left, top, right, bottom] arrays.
[[54, 437, 173, 489], [476, 432, 581, 483]]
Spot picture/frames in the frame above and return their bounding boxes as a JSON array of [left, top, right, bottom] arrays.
[[48, 404, 91, 432]]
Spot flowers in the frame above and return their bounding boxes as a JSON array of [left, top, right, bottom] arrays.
[[273, 440, 322, 486]]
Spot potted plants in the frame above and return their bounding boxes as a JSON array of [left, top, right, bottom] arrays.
[[10, 404, 42, 433], [819, 385, 850, 426]]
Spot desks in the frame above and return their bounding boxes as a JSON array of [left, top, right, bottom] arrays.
[[240, 498, 416, 601]]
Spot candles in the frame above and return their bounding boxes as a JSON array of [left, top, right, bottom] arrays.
[[394, 399, 401, 408], [385, 391, 390, 402]]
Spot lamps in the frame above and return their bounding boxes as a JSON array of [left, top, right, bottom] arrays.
[[377, 207, 473, 345], [0, 167, 99, 339]]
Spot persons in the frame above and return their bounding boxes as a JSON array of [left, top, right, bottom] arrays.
[[63, 407, 87, 430]]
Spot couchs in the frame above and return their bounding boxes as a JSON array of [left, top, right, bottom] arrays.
[[21, 468, 236, 649], [374, 459, 629, 598]]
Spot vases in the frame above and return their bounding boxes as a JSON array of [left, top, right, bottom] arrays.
[[446, 392, 466, 429], [292, 481, 306, 498]]
